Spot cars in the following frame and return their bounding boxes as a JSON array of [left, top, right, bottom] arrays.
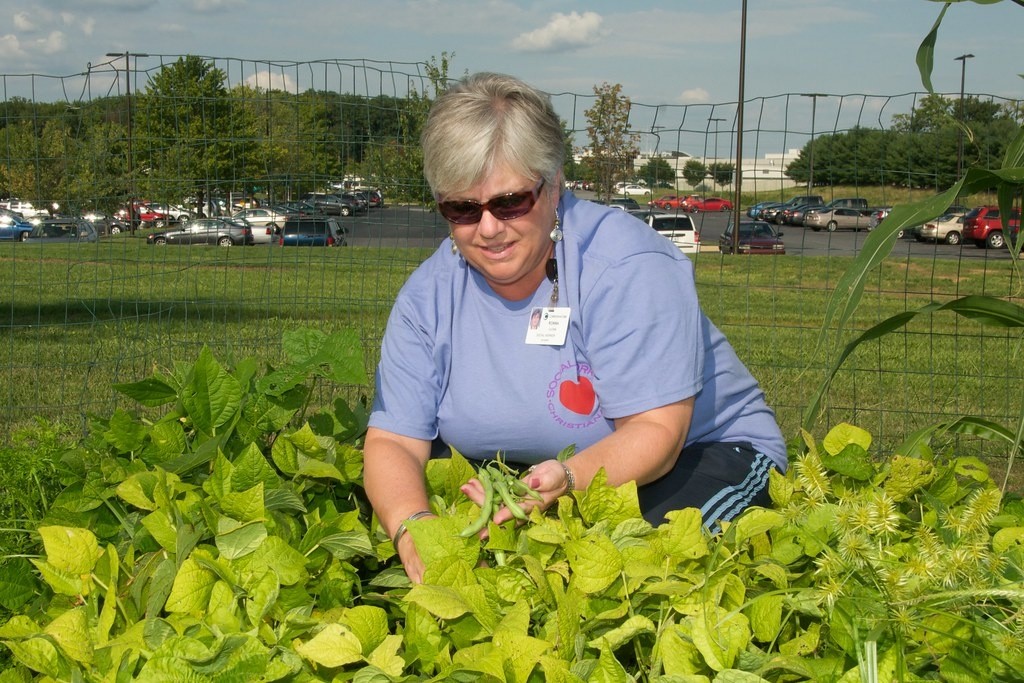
[[719, 222, 785, 255], [687, 198, 733, 214], [274, 216, 349, 247], [0, 187, 385, 244], [746, 195, 973, 242], [23, 218, 101, 244], [564, 180, 633, 194], [802, 207, 871, 233], [681, 194, 703, 210], [618, 185, 653, 197], [585, 198, 640, 211], [660, 196, 685, 211], [147, 218, 254, 247], [625, 210, 667, 222], [654, 183, 674, 189], [919, 213, 969, 245], [648, 196, 675, 209]]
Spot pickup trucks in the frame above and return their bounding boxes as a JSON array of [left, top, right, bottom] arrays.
[[825, 198, 893, 212]]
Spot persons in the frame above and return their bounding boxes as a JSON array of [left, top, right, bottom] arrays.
[[364, 73, 788, 585]]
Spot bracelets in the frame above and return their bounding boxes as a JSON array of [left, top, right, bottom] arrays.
[[393, 510, 434, 553], [561, 463, 575, 496]]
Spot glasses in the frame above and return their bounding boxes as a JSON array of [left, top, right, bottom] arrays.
[[438, 176, 546, 224]]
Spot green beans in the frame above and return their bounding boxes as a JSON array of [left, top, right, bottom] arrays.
[[457, 463, 545, 567]]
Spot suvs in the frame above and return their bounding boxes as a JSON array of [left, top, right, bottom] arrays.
[[644, 213, 701, 253], [964, 205, 1024, 249]]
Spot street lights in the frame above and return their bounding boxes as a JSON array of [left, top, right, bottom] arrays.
[[803, 93, 830, 195], [954, 52, 974, 184], [707, 118, 729, 195], [105, 51, 150, 235], [651, 126, 666, 192]]
